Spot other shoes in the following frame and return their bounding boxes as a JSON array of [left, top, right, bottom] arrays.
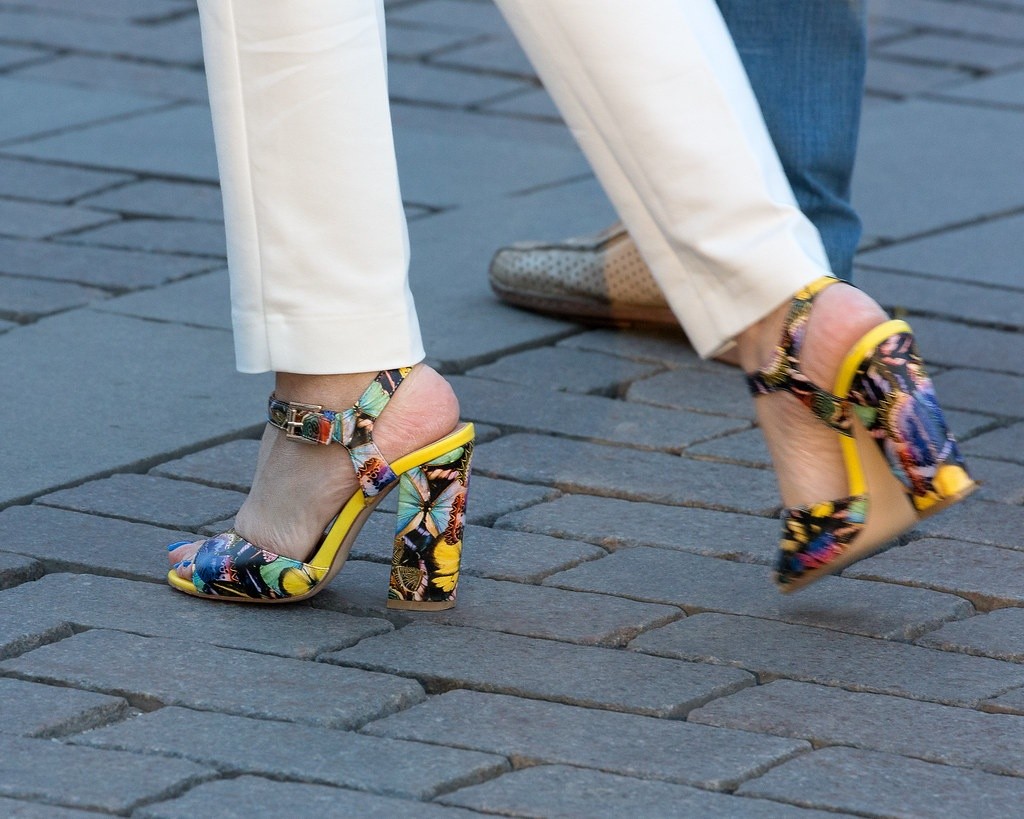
[[488, 227, 681, 324]]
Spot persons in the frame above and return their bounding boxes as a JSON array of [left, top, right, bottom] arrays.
[[163, 0, 980, 613], [487, 0, 864, 325]]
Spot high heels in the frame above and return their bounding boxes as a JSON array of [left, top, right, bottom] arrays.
[[745, 275, 980, 595], [167, 365, 474, 612]]
